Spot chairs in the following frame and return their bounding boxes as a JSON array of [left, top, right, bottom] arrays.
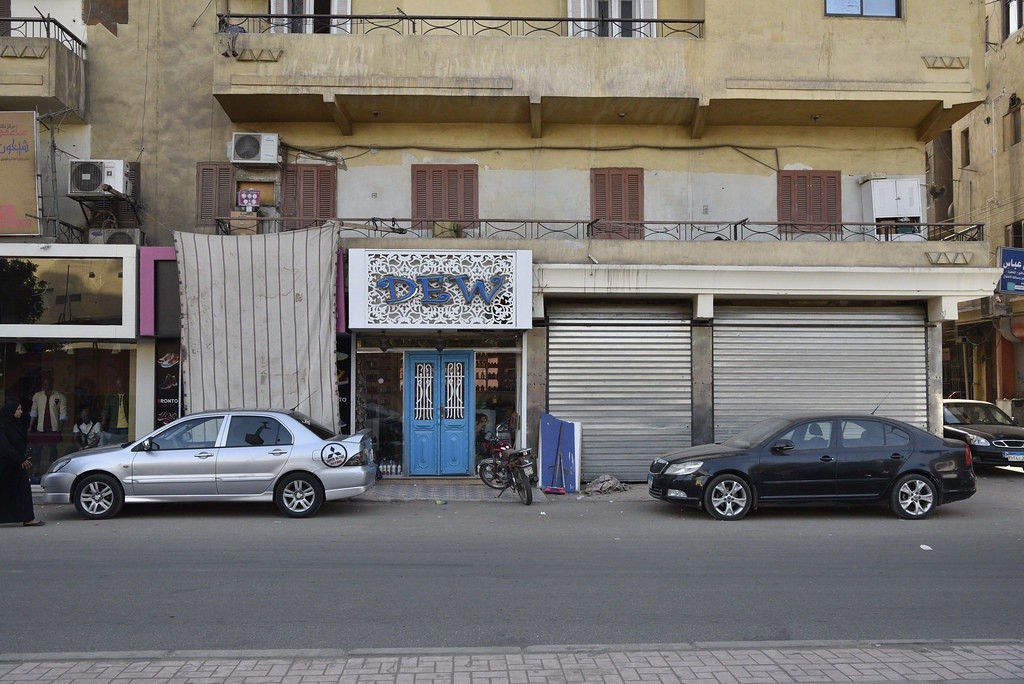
[[968, 412, 980, 423], [806, 424, 826, 448]]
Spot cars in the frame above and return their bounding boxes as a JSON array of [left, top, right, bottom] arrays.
[[646, 411, 978, 522], [41, 407, 378, 520], [943, 399, 1024, 472]]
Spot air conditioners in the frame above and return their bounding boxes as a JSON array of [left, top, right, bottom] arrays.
[[89, 228, 141, 245], [68, 159, 132, 196], [230, 132, 282, 166], [981, 294, 1006, 316]]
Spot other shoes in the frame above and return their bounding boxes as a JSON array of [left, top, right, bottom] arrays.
[[23, 520, 45, 528], [31, 467, 39, 477]]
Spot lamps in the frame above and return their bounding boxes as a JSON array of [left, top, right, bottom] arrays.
[[379, 331, 390, 352], [436, 330, 445, 352]]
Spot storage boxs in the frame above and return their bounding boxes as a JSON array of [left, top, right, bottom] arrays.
[[433, 222, 463, 238], [228, 189, 261, 234], [879, 221, 896, 234]]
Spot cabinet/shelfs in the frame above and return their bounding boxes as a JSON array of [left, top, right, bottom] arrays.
[[399, 353, 516, 404], [861, 178, 928, 241]]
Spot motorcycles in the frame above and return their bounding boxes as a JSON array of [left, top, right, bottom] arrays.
[[477, 418, 535, 506]]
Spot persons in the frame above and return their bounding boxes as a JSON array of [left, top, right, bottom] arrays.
[[0, 400, 46, 526], [28, 375, 68, 485], [73, 406, 101, 450], [101, 379, 129, 447]]
